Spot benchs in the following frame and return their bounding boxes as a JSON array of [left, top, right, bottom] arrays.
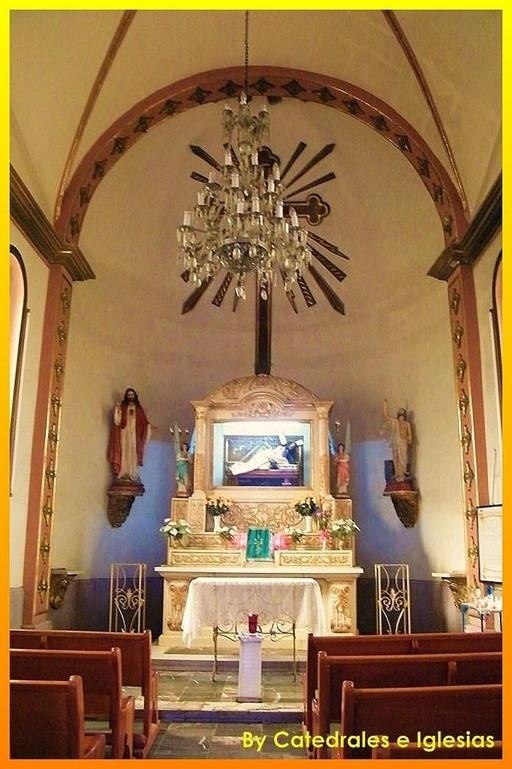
[[5, 622, 164, 761], [296, 628, 503, 756]]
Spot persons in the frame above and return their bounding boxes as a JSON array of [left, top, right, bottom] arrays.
[[174, 442, 195, 498], [382, 398, 412, 480], [228, 440, 297, 475], [109, 388, 161, 482], [333, 443, 351, 495]]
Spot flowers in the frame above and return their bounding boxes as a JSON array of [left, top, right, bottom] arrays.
[[157, 494, 361, 548]]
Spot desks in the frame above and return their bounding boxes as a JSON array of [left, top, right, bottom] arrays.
[[176, 577, 323, 685]]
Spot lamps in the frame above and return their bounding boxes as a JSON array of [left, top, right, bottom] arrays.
[[180, 15, 315, 303]]
